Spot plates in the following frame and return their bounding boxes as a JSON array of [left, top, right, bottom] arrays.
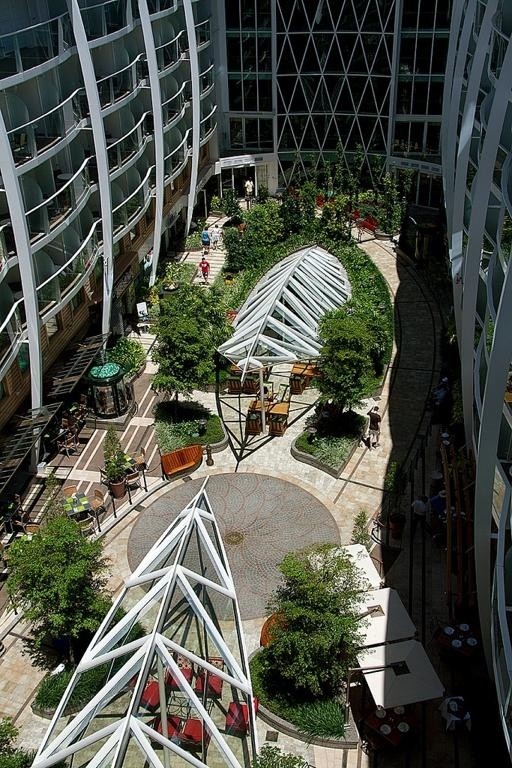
[[376, 706, 410, 734], [445, 624, 477, 648]]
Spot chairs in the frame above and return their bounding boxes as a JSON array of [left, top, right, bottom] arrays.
[[63, 446, 147, 539], [131, 651, 258, 751], [1, 492, 40, 562], [228, 376, 305, 437], [364, 620, 478, 748], [57, 393, 88, 459]]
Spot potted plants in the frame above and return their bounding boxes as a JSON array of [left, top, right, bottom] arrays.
[[382, 460, 406, 540], [104, 421, 126, 498]]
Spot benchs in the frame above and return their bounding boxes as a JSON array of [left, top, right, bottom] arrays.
[[160, 444, 203, 481], [260, 610, 289, 646]]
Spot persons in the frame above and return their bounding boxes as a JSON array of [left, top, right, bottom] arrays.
[[199, 258, 210, 284], [428, 376, 453, 425], [202, 227, 211, 256], [210, 224, 219, 251], [367, 406, 381, 450], [244, 177, 254, 198], [409, 471, 448, 543]]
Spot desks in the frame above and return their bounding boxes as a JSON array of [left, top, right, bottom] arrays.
[[47, 427, 67, 445], [290, 363, 319, 386], [230, 363, 268, 381]]
